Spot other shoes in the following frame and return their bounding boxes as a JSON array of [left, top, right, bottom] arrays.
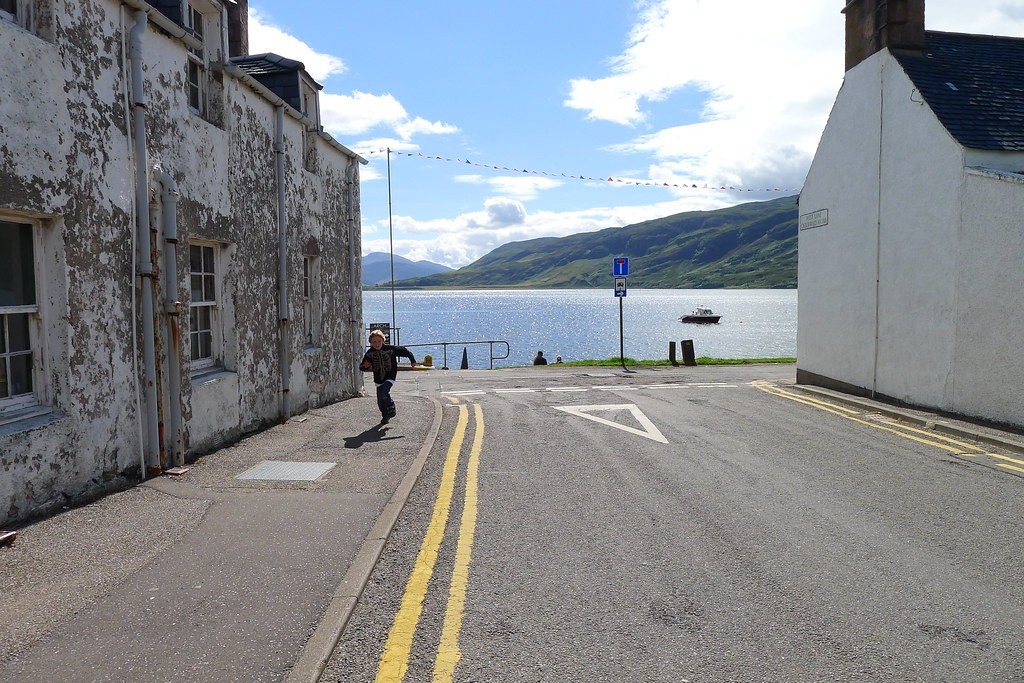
[[381, 416, 390, 424], [389, 404, 396, 417]]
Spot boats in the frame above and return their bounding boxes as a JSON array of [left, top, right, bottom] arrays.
[[677, 304, 724, 324]]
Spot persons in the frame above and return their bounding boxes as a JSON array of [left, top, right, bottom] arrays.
[[360, 329, 417, 423], [555, 356, 564, 364], [534, 350, 547, 365]]
